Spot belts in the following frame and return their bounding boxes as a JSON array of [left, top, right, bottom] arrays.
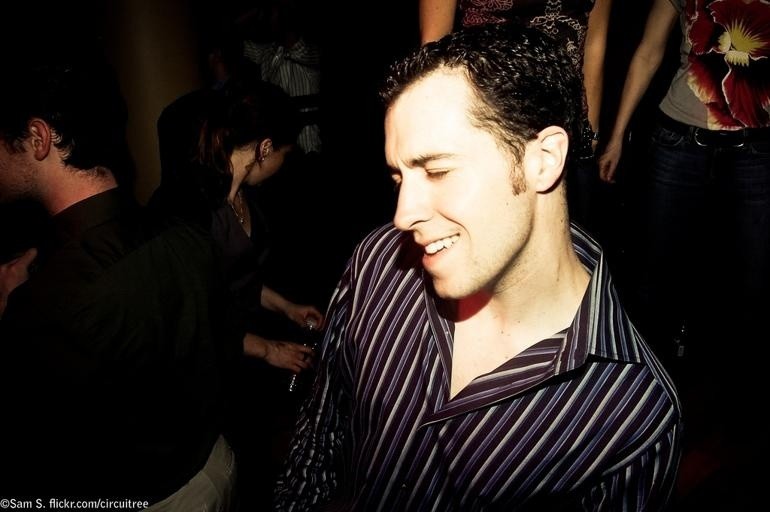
[[661, 114, 763, 150]]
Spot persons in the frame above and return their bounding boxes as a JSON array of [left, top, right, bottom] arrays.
[[0, 0, 770, 512]]
